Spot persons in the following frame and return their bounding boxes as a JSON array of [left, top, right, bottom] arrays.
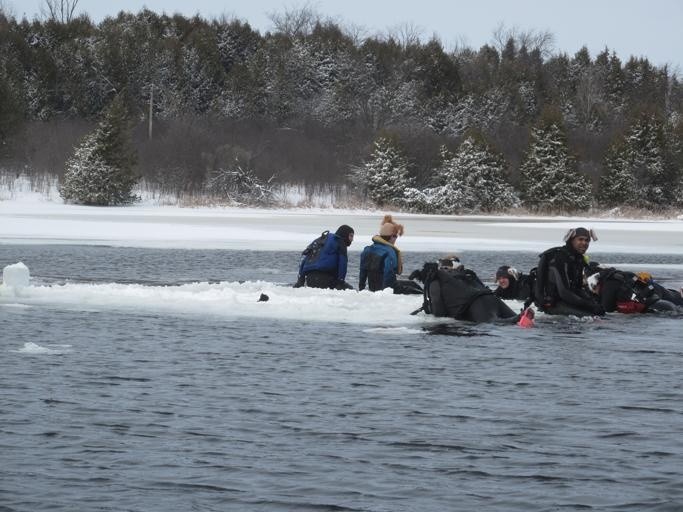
[[435, 255, 462, 271], [587, 261, 682, 317], [409, 263, 536, 330], [490, 265, 533, 301], [534, 227, 606, 319], [356, 214, 405, 294], [293, 224, 355, 291]]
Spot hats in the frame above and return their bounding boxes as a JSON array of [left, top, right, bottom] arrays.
[[335, 225, 354, 246], [495, 266, 518, 291], [379, 215, 403, 237], [563, 228, 597, 256]]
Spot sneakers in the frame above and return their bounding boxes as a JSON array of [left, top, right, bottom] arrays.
[[519, 307, 534, 328]]
